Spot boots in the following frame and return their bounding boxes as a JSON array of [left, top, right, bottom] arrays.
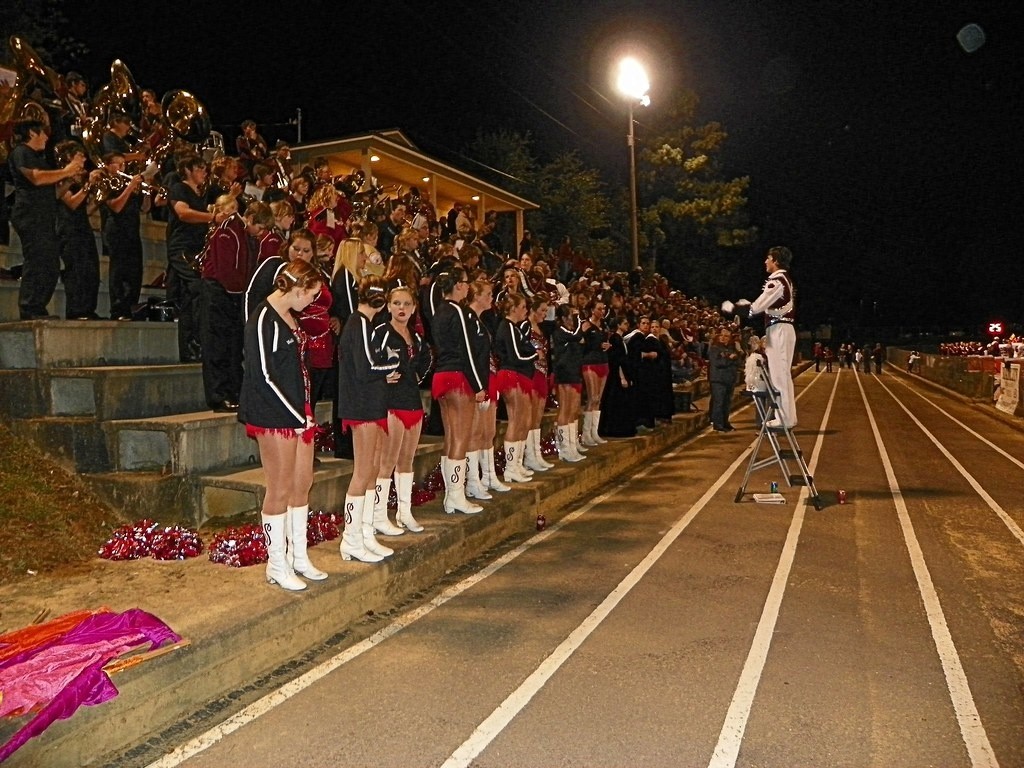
[[394, 472, 424, 532], [574, 419, 589, 453], [372, 478, 404, 535], [556, 422, 586, 462], [524, 428, 554, 471], [261, 503, 328, 590], [441, 455, 483, 514], [340, 489, 393, 562], [466, 446, 511, 499], [504, 440, 534, 483], [581, 411, 608, 447]]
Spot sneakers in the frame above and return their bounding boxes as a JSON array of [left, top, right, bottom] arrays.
[[762, 418, 798, 428]]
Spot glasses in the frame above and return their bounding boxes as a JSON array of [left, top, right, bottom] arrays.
[[454, 280, 470, 284]]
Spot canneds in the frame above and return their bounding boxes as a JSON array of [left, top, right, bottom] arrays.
[[537, 514, 545, 531], [839, 489, 846, 504], [770, 481, 778, 493]]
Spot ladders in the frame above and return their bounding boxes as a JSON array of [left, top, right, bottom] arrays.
[[734, 359, 821, 512]]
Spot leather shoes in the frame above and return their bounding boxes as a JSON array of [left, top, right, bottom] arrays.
[[213, 400, 239, 414]]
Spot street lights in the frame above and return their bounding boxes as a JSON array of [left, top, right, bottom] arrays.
[[618, 56, 650, 272]]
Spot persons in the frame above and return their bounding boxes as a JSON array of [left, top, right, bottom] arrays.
[[722, 245, 799, 427], [904, 336, 1024, 375], [337, 274, 400, 563], [815, 340, 885, 374], [0, 36, 769, 535], [239, 259, 328, 591]]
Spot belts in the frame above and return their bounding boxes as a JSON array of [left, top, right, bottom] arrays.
[[767, 320, 793, 327]]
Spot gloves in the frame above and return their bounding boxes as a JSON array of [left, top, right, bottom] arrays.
[[736, 298, 752, 306], [722, 300, 734, 313]]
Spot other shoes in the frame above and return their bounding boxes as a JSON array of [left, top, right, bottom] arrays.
[[713, 424, 736, 432], [30, 314, 61, 320]]
